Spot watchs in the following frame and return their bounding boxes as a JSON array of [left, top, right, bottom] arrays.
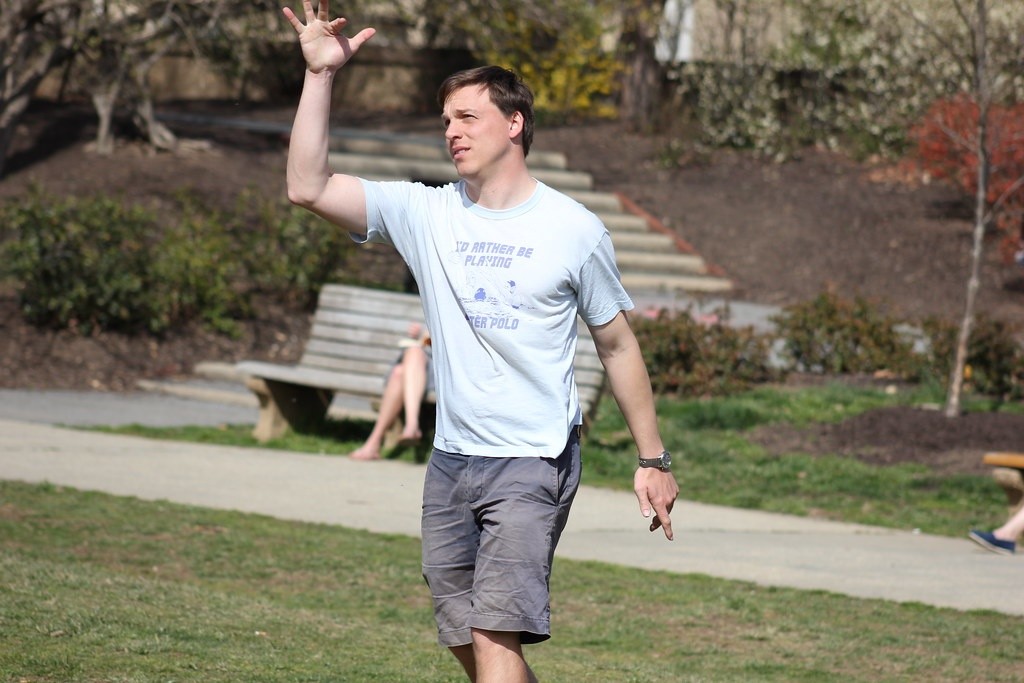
[[638, 450, 673, 472]]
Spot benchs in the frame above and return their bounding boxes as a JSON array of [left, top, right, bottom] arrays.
[[237, 282, 605, 443]]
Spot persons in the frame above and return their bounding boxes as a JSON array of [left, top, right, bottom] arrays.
[[282, 1, 680, 683], [350, 321, 437, 462], [968, 505, 1024, 555]]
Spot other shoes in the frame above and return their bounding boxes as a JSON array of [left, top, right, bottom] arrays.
[[352, 444, 381, 459], [968, 529, 1016, 555], [396, 430, 422, 445]]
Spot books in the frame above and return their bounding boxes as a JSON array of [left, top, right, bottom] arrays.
[[397, 332, 431, 349]]
